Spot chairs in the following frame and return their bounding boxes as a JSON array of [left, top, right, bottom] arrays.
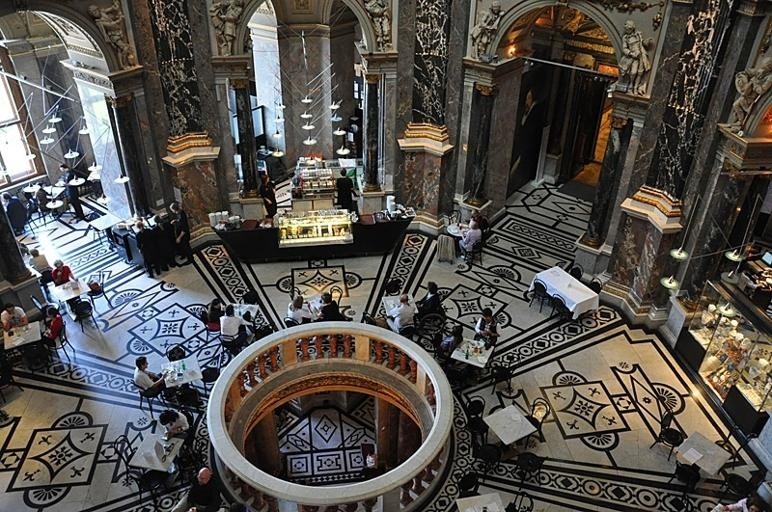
[[529, 263, 603, 328], [361, 310, 552, 511], [0, 269, 112, 404], [650, 409, 767, 512], [114, 308, 241, 510], [436, 210, 492, 269]]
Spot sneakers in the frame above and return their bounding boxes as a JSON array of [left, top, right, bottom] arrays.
[[148, 264, 175, 278], [461, 255, 467, 259]]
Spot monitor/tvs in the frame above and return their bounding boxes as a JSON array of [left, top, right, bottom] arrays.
[[761, 250, 772, 268]]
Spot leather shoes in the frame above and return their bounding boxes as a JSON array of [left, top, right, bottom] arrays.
[[185, 259, 194, 264]]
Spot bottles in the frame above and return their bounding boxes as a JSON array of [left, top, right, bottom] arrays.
[[239, 305, 241, 316], [465, 349, 469, 360], [181, 360, 185, 370]]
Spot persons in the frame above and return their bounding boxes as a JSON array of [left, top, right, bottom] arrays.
[[287, 296, 303, 325], [470, 1, 505, 63], [219, 304, 254, 357], [0, 249, 78, 347], [336, 168, 353, 211], [510, 88, 538, 192], [459, 220, 482, 260], [723, 496, 767, 512], [717, 56, 772, 134], [2, 164, 85, 236], [474, 308, 501, 346], [88, 2, 136, 71], [440, 325, 463, 355], [134, 356, 168, 399], [471, 209, 491, 240], [206, 298, 225, 331], [159, 409, 190, 438], [618, 20, 653, 96], [391, 294, 415, 334], [415, 282, 441, 322], [259, 174, 277, 219], [188, 467, 223, 512], [365, 0, 393, 53], [135, 202, 194, 278], [316, 292, 346, 321], [221, 0, 243, 57]]
